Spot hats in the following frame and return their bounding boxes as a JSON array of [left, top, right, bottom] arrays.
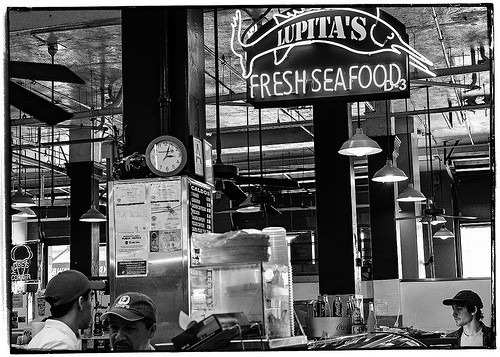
[[99, 292, 157, 322], [443, 290, 483, 309], [44, 270, 105, 306]]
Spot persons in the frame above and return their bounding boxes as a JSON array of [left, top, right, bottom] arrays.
[[100, 292, 157, 351], [442, 289, 496, 350], [25, 270, 105, 350]]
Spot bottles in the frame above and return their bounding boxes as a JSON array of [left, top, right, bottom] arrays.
[[317, 294, 342, 316], [347, 294, 376, 333]]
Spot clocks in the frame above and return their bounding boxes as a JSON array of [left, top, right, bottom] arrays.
[[146, 136, 187, 177]]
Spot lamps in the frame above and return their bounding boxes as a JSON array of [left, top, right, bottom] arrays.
[[79, 119, 108, 222], [372, 101, 409, 183], [433, 141, 456, 239], [395, 99, 427, 202], [419, 79, 447, 225], [11, 109, 36, 207], [336, 102, 383, 156]]
